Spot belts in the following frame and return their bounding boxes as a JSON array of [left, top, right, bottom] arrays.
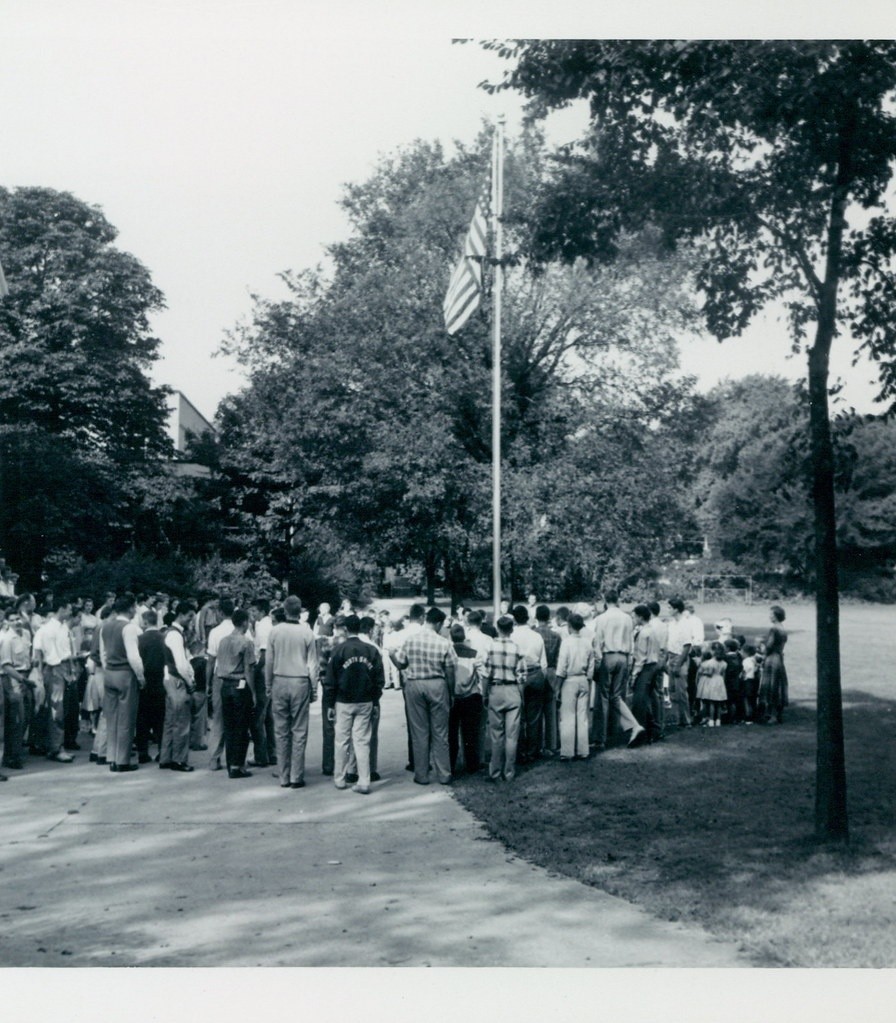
[[490, 681, 518, 686], [521, 667, 541, 673]]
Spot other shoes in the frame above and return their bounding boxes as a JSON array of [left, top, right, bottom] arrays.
[[428, 764, 433, 770], [371, 771, 380, 782], [345, 773, 358, 783], [110, 761, 138, 773], [322, 771, 333, 776], [406, 764, 415, 772], [579, 754, 588, 760], [0, 774, 7, 781], [716, 719, 721, 727], [745, 721, 753, 725], [190, 743, 209, 752], [247, 759, 260, 766], [627, 726, 644, 747], [230, 767, 252, 779], [707, 720, 715, 728], [282, 780, 305, 788], [560, 754, 573, 762], [0, 724, 153, 769], [160, 759, 193, 771]]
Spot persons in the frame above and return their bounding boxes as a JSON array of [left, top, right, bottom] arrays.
[[0, 592, 788, 794]]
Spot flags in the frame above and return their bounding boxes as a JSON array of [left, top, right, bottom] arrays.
[[444, 129, 494, 336]]
